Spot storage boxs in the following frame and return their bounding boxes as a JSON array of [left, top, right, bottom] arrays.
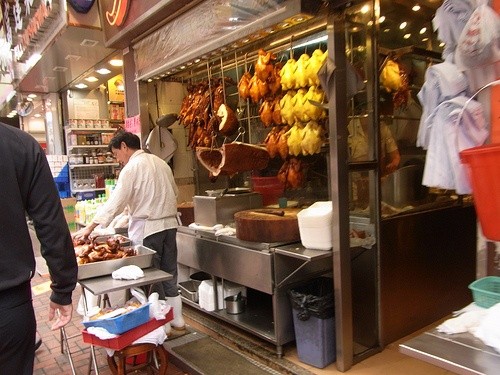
[[82, 306, 175, 351], [197, 279, 247, 312], [82, 301, 152, 334], [90, 172, 105, 188], [60, 197, 78, 233]]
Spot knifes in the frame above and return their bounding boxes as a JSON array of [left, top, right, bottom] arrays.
[[254, 210, 284, 216]]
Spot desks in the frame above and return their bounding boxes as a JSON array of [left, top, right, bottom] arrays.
[[60, 265, 174, 375]]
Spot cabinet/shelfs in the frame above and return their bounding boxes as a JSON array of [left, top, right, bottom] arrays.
[[64, 124, 119, 198]]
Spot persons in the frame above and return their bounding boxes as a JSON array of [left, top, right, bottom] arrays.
[[0, 121, 79, 375], [347, 101, 400, 201], [70, 131, 187, 329]]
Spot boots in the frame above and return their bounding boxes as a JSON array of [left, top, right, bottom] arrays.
[[163, 323, 174, 336], [165, 294, 187, 330]]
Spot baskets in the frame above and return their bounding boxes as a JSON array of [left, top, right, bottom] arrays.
[[469, 274, 500, 308]]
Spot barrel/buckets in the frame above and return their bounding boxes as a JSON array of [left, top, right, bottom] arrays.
[[454, 79, 500, 242]]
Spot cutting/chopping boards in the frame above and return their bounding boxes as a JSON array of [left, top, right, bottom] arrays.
[[235, 208, 301, 242]]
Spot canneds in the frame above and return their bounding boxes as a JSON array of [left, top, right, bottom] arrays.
[[70, 150, 113, 164]]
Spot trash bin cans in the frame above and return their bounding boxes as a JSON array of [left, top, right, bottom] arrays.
[[287, 277, 336, 368]]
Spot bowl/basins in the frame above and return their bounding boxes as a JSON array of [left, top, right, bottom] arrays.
[[252, 177, 285, 204], [225, 296, 246, 314]]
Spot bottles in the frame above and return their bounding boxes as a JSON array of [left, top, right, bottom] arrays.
[[105, 175, 118, 197], [75, 194, 107, 224]]
